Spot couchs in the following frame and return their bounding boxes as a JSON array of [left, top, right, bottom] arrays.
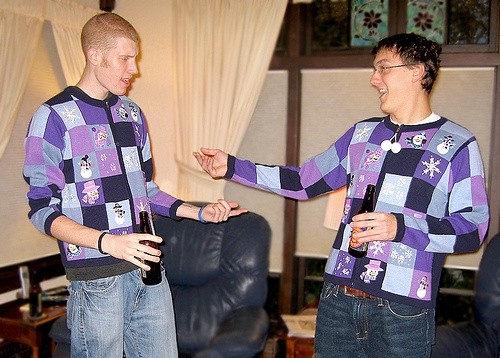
[[154, 211, 270, 358], [431, 233, 500, 358]]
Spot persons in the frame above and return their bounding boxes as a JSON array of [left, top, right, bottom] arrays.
[[192, 33, 490, 358], [21, 13, 248, 358]]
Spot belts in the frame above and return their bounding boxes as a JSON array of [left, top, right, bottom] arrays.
[[340, 285, 375, 299]]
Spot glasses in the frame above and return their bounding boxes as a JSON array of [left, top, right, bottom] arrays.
[[374, 63, 417, 75]]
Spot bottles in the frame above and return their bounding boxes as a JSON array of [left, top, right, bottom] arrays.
[[139, 211, 162, 286], [348, 184, 376, 258], [28, 270, 42, 317]]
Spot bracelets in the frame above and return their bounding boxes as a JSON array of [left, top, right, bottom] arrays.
[[198, 205, 206, 223], [98, 232, 108, 255]]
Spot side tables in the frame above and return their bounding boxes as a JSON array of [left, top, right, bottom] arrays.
[[286, 303, 318, 358], [0, 286, 70, 358]]
